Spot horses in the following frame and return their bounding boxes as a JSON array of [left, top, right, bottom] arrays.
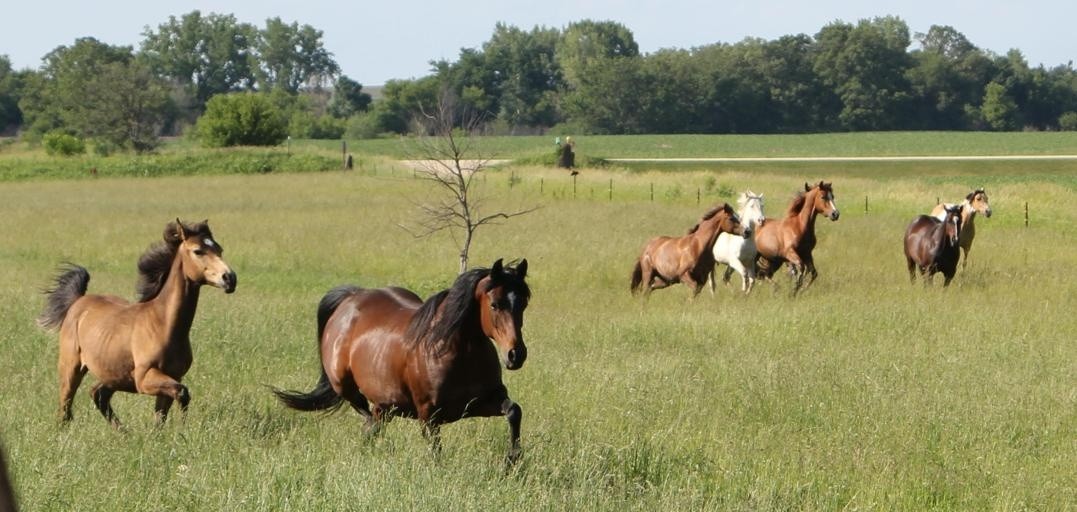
[[708, 188, 764, 299], [630, 203, 753, 301], [723, 181, 840, 299], [904, 204, 964, 289], [34, 217, 238, 433], [931, 186, 992, 266], [262, 258, 532, 477]]
